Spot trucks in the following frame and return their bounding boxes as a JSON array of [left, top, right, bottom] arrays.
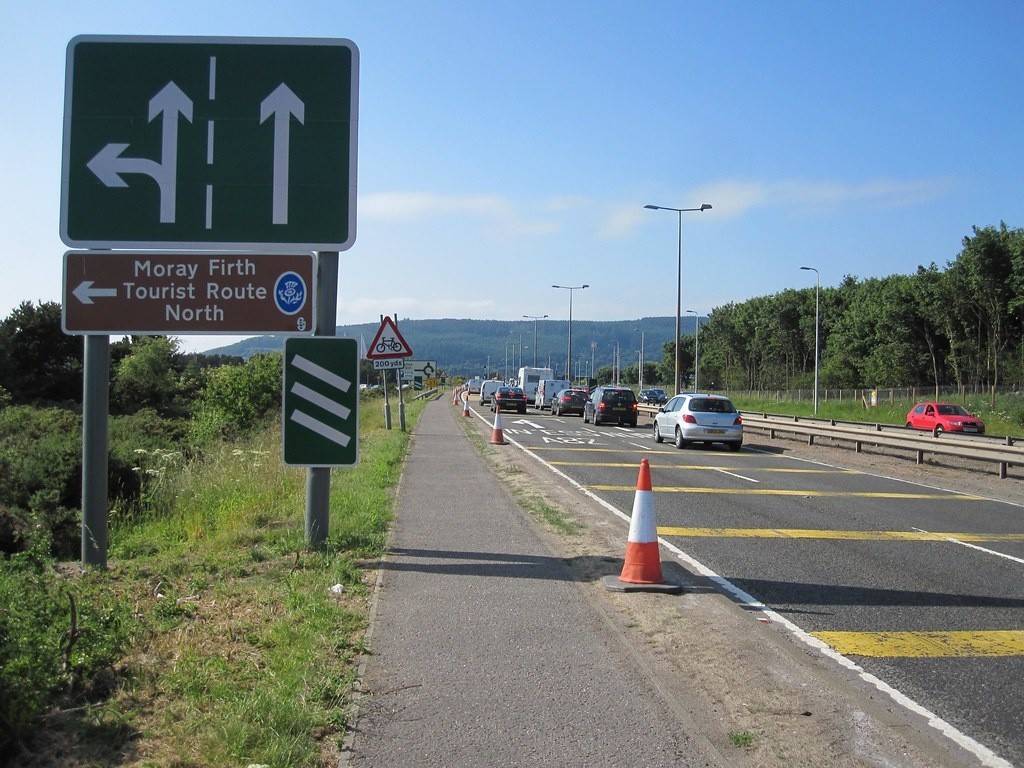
[[467, 379, 506, 406], [515, 366, 570, 410]]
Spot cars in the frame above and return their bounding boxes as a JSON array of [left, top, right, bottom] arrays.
[[906, 402, 986, 435], [550, 386, 638, 428], [490, 386, 527, 415], [636, 388, 743, 452]]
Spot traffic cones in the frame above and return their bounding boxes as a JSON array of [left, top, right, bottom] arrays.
[[462, 395, 473, 418], [452, 386, 464, 405], [601, 458, 680, 594], [489, 406, 510, 445]]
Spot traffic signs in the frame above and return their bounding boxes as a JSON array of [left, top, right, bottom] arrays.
[[62, 250, 318, 338]]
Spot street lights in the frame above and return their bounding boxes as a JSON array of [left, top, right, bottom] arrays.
[[686, 310, 698, 394], [608, 340, 620, 388], [523, 315, 549, 368], [645, 203, 713, 397], [506, 331, 528, 381], [634, 329, 644, 391], [800, 266, 819, 415], [552, 285, 589, 385]]
